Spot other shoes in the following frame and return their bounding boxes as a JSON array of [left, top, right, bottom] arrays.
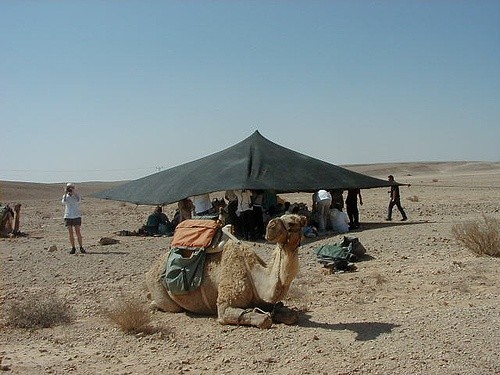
[[400, 217, 407, 221], [80, 246, 85, 253], [70, 248, 75, 254], [386, 218, 392, 221]]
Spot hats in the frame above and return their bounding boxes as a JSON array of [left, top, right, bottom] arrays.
[[65, 183, 76, 190]]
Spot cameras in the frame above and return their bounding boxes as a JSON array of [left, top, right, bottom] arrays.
[[66, 187, 72, 193]]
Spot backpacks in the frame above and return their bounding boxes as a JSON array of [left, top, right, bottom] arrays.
[[172, 219, 219, 248], [166, 248, 206, 295]]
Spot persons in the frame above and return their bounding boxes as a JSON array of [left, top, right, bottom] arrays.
[[61, 182, 85, 254], [388, 175, 408, 222], [144, 187, 363, 243]]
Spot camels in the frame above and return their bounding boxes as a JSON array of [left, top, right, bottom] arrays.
[[0, 202, 22, 238], [146, 214, 307, 329]]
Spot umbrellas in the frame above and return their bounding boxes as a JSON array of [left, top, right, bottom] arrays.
[[84, 130, 411, 207]]
